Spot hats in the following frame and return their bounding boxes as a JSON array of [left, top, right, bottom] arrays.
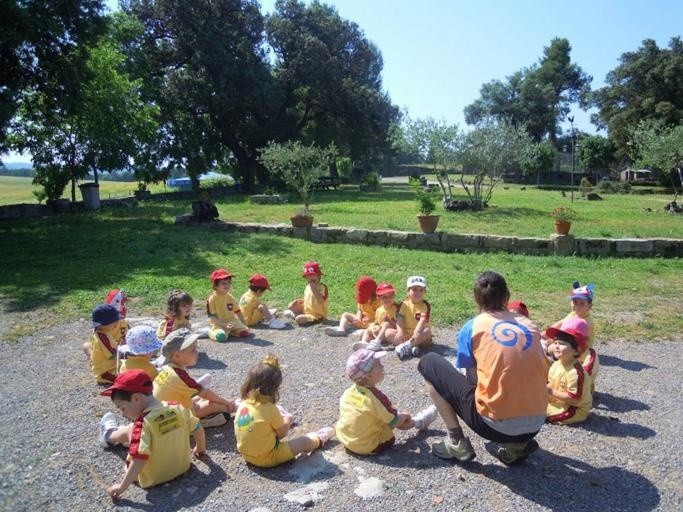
[[93, 289, 127, 325], [100, 370, 152, 396], [546, 319, 588, 352], [356, 276, 395, 304], [346, 349, 387, 379], [210, 269, 234, 283], [407, 276, 426, 288], [568, 282, 594, 300], [304, 262, 322, 276], [250, 275, 272, 291], [118, 326, 203, 357]]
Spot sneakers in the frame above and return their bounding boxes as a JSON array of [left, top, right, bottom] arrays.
[[325, 327, 345, 336], [499, 440, 538, 464], [416, 405, 438, 430], [100, 412, 117, 448], [432, 437, 476, 462], [316, 427, 335, 447], [269, 310, 295, 329], [352, 340, 419, 359], [200, 412, 230, 428]]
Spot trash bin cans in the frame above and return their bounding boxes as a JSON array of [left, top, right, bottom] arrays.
[[79, 182, 101, 210]]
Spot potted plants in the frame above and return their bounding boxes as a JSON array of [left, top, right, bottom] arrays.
[[409, 176, 443, 233]]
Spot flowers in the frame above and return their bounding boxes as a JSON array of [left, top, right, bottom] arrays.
[[550, 206, 574, 223]]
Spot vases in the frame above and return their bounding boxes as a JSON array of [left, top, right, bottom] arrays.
[[290, 216, 313, 226], [552, 220, 571, 235]]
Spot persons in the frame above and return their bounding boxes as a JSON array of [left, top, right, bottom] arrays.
[[540, 280, 601, 426], [82, 289, 238, 498], [334, 350, 437, 454], [205, 262, 328, 341], [234, 359, 337, 467], [415, 271, 551, 467], [326, 275, 432, 358]]
